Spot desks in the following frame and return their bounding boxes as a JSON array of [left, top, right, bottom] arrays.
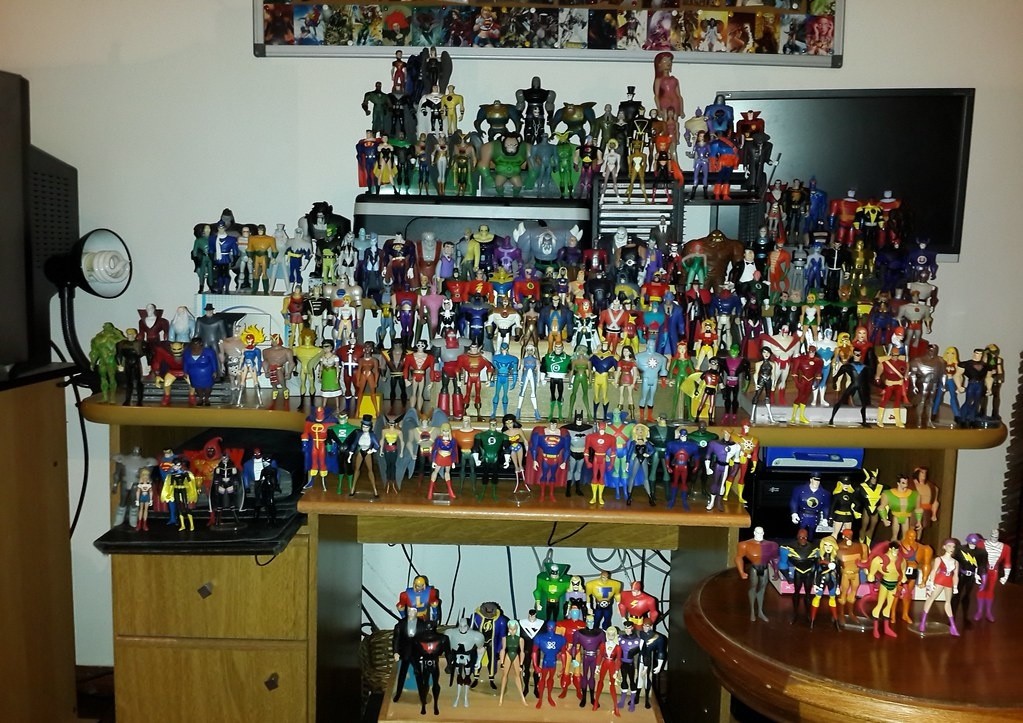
[[682, 565, 1023, 723], [0, 362, 78, 723]]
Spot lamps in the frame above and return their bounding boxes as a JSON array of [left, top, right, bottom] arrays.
[[43, 227, 133, 394]]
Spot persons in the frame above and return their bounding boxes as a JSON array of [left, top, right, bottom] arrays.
[[356, 45, 778, 203], [789, 464, 939, 546], [189, 200, 463, 418], [890, 530, 933, 624], [110, 436, 283, 533], [86, 302, 295, 412], [391, 563, 668, 716], [449, 219, 745, 429], [298, 408, 759, 510], [714, 173, 1007, 428], [867, 541, 908, 639], [973, 529, 1011, 622], [808, 537, 842, 631], [778, 529, 820, 626], [951, 534, 988, 629], [836, 529, 868, 627], [735, 526, 780, 622], [919, 538, 960, 636]]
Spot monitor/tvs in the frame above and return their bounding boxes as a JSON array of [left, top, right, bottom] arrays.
[[714, 87, 975, 263], [0, 70, 82, 379]]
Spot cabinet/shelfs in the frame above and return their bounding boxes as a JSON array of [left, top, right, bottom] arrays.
[[79, 395, 1007, 723]]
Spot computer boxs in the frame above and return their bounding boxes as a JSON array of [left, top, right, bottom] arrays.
[[740, 466, 870, 542]]
